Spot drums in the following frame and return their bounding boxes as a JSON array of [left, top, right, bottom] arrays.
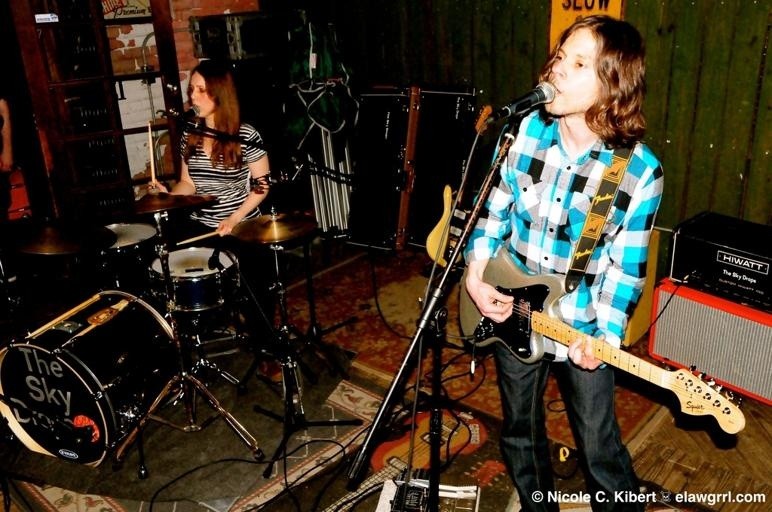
[[0, 289, 179, 469], [149, 247, 242, 312], [79, 223, 161, 291]]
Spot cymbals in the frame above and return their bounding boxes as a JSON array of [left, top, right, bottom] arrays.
[[115, 190, 216, 214], [232, 212, 316, 246], [5, 217, 119, 256]]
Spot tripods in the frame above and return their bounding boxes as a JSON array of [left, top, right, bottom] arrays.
[[292, 122, 361, 382], [252, 205, 366, 478], [111, 185, 268, 467]]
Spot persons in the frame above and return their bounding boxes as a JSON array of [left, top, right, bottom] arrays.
[[147, 58, 271, 242], [465, 15, 663, 512]]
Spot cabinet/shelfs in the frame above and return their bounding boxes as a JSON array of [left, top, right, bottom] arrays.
[[9, 0, 182, 221]]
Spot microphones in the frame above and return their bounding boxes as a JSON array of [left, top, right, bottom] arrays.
[[487, 80, 555, 125], [173, 102, 201, 131]]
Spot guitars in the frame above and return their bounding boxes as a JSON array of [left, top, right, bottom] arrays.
[[426, 105, 492, 270], [459, 248, 746, 436], [321, 409, 489, 512]]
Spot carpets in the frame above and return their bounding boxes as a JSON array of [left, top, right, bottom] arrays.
[[0, 253, 720, 512]]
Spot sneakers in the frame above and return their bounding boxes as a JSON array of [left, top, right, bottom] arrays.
[[256, 361, 285, 382]]
